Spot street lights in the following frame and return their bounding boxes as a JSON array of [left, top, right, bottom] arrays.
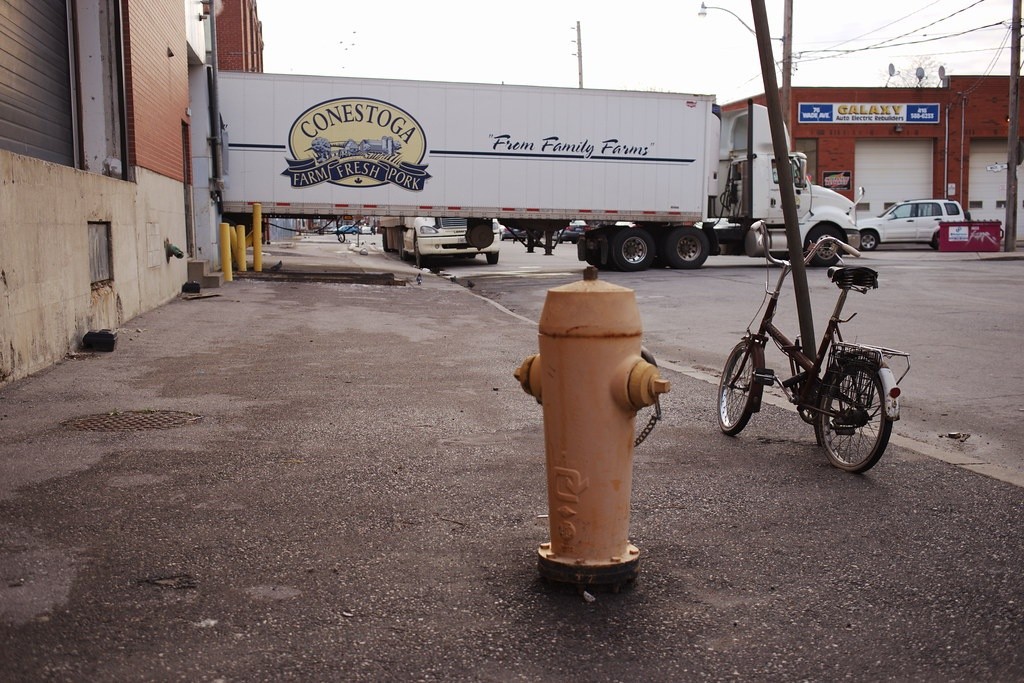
[[697, 1, 793, 144]]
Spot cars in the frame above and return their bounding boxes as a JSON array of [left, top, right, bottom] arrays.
[[331, 225, 374, 236], [501, 220, 591, 244]]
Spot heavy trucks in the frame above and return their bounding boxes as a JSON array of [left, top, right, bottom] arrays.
[[212, 71, 866, 273], [382, 211, 503, 268]]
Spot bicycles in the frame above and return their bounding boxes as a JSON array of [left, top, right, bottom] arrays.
[[717, 219, 911, 475]]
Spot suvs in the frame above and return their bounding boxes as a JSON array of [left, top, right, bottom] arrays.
[[856, 198, 969, 252]]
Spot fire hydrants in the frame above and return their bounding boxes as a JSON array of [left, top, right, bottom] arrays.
[[513, 265, 670, 595]]
